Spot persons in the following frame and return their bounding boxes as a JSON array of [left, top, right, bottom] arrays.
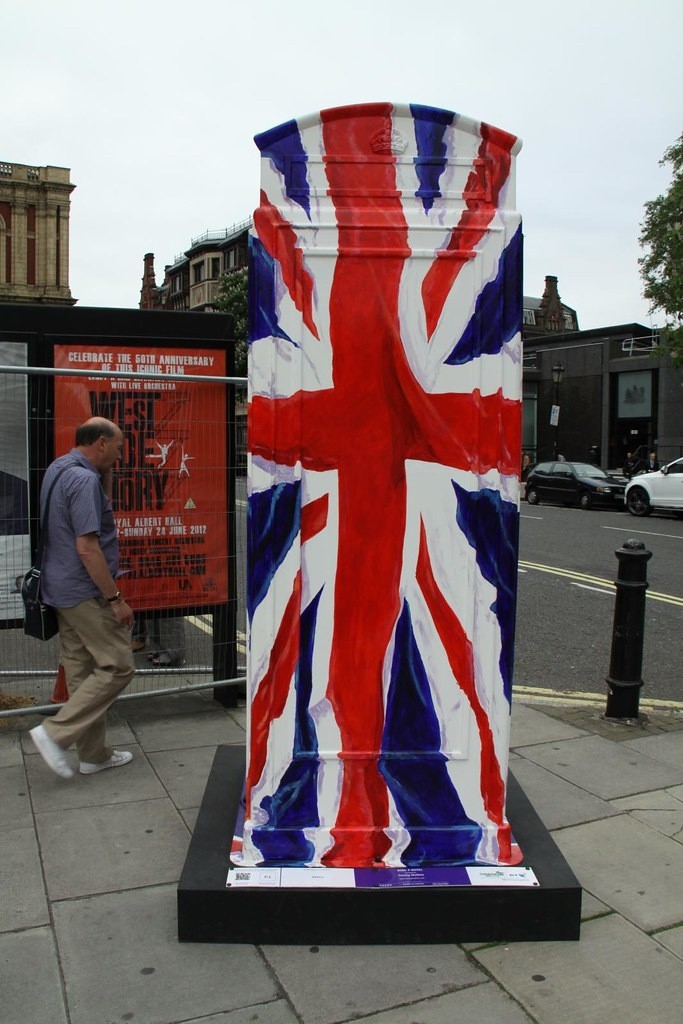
[[645, 452, 658, 473], [27, 416, 136, 778], [623, 452, 633, 479]]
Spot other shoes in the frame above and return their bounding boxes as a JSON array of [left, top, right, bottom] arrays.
[[152, 658, 163, 666], [133, 640, 145, 653], [147, 653, 155, 660]]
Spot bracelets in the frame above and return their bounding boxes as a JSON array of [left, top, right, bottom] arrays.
[[112, 596, 124, 607]]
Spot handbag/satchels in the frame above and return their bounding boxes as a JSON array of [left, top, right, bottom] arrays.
[[21, 566, 59, 641]]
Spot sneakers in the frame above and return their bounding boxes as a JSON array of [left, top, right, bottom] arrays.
[[79, 749, 132, 774], [28, 724, 74, 779]]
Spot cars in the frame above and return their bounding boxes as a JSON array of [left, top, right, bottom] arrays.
[[624, 457, 683, 518], [525, 460, 628, 513]]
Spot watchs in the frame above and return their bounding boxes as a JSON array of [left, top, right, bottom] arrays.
[[107, 591, 121, 603]]
